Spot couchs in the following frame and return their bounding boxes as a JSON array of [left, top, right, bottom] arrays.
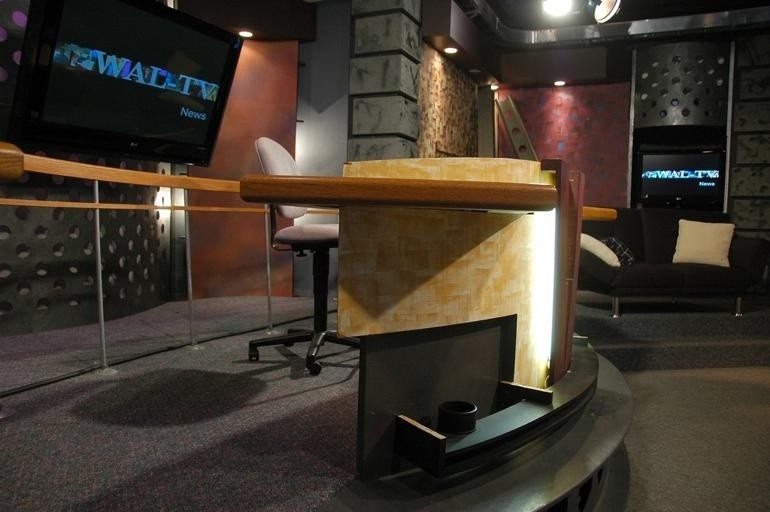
[[580, 207, 769, 317]]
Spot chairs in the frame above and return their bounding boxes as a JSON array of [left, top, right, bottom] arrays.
[[250, 134, 359, 374]]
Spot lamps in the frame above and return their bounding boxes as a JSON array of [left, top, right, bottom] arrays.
[[541, 0, 621, 23]]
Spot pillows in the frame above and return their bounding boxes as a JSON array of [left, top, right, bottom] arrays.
[[580, 232, 635, 267], [673, 219, 735, 268]]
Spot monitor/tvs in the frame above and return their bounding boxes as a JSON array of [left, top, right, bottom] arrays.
[[6, 0, 244, 168], [635, 149, 726, 207]]
